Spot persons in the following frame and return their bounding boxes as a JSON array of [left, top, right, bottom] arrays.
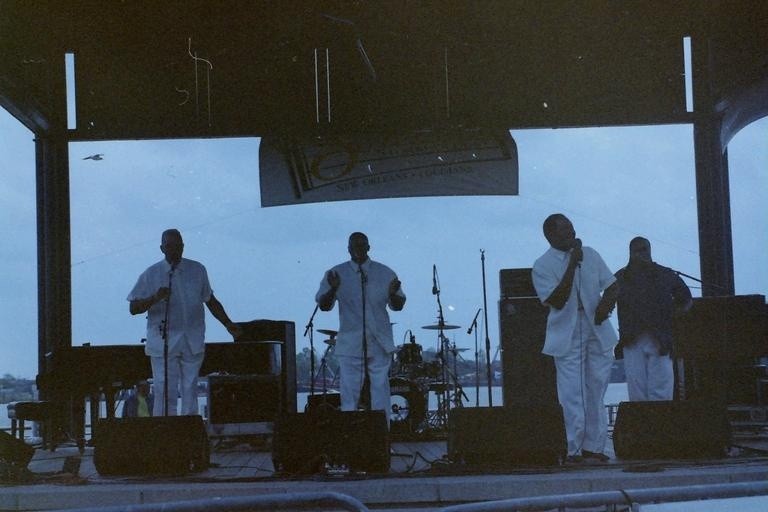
[[126, 228, 246, 419], [530, 214, 621, 468], [123, 376, 157, 419], [613, 236, 694, 402], [315, 231, 407, 455]]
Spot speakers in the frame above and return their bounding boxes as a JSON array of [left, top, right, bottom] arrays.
[[613, 400, 733, 462], [207, 372, 285, 423], [272, 410, 392, 478], [233, 320, 298, 413], [445, 405, 568, 472], [1, 428, 36, 482], [92, 414, 211, 479], [674, 292, 768, 404], [499, 268, 538, 295], [498, 296, 560, 405]]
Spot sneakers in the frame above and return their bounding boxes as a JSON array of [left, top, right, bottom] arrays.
[[583, 451, 609, 462], [564, 456, 585, 466]]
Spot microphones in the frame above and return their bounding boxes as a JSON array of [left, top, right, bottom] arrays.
[[636, 255, 657, 269], [432, 265, 438, 295], [572, 237, 583, 268], [170, 250, 179, 276], [466, 308, 481, 334], [352, 244, 365, 262]]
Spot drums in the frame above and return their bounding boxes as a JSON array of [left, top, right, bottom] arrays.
[[401, 364, 426, 378], [396, 343, 423, 364], [424, 361, 443, 377], [388, 377, 425, 432]]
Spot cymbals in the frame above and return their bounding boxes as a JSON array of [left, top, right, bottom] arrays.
[[316, 329, 339, 336], [447, 347, 469, 351], [324, 339, 336, 345], [421, 325, 461, 330]]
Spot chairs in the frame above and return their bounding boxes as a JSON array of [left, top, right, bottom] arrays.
[[7, 374, 57, 452]]
